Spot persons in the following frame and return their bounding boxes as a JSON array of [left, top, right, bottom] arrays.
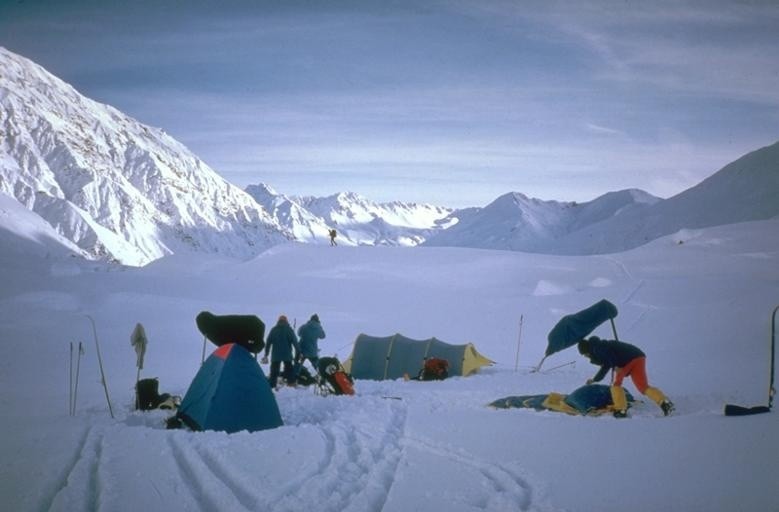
[[576, 336, 675, 420], [263, 313, 300, 389], [293, 315, 327, 369], [328, 228, 336, 244]]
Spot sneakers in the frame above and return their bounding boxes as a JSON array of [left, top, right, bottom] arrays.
[[614, 408, 626, 417], [661, 399, 674, 416]]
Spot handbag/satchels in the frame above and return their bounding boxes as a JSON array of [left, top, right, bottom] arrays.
[[331, 368, 354, 395], [135, 379, 157, 408], [417, 357, 447, 380]]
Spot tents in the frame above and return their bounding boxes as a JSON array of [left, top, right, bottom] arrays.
[[339, 331, 504, 380], [175, 339, 284, 432]]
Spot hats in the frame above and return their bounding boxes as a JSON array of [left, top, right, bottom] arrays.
[[578, 340, 589, 354]]
